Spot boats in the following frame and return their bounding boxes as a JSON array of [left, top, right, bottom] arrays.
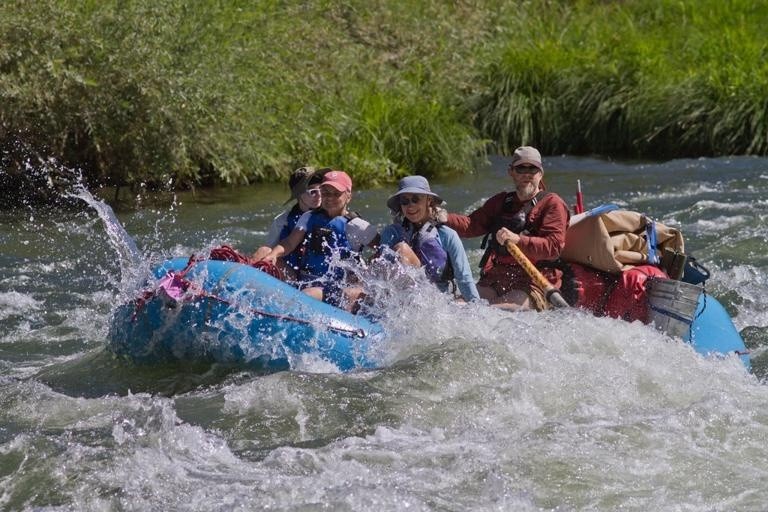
[[105, 257, 753, 382]]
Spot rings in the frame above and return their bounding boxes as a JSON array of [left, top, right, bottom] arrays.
[[500, 234, 504, 238]]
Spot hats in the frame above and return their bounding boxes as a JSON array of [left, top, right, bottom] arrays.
[[386, 175, 441, 209], [318, 170, 352, 193], [511, 146, 542, 172], [281, 166, 331, 206]]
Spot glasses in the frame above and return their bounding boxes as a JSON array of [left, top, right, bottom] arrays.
[[515, 165, 540, 174], [398, 197, 419, 205], [305, 188, 321, 198]]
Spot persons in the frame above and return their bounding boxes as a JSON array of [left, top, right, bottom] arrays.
[[250, 166, 329, 265], [260, 170, 381, 303], [428, 146, 569, 310], [335, 175, 482, 316]]
